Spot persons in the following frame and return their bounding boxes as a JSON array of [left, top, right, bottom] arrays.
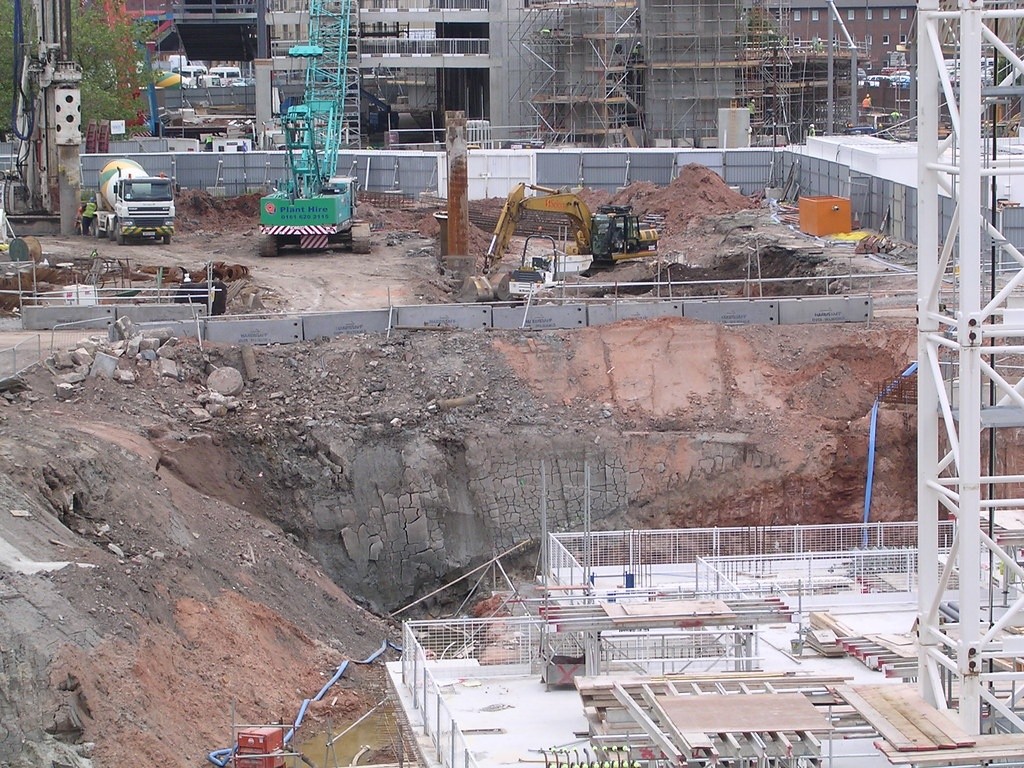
[[890, 111, 903, 122], [809, 124, 814, 136], [749, 99, 755, 123], [633, 42, 643, 61], [816, 37, 824, 52], [863, 94, 871, 108]]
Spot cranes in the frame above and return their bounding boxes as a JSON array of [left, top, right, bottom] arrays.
[[373, 0, 1024, 768]]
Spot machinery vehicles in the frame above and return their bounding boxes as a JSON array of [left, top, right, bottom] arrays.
[[456, 191, 663, 308], [257, 0, 374, 256], [91, 158, 175, 245]]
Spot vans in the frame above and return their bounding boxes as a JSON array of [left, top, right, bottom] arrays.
[[171, 59, 242, 89]]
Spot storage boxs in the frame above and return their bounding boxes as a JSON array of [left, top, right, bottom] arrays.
[[798, 195, 852, 237]]
